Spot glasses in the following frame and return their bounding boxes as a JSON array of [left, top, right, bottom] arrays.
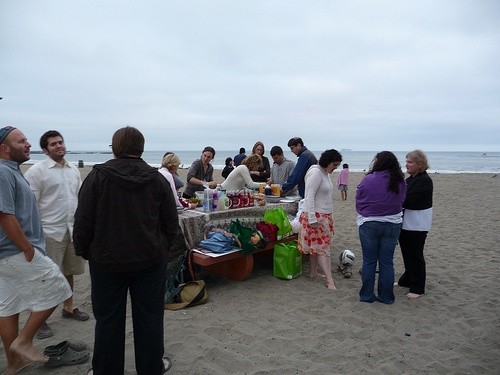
[[291, 146, 295, 150]]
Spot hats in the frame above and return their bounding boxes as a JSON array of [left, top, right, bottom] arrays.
[[0, 126, 17, 145]]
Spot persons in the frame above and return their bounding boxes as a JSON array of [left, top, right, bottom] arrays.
[[249, 142, 270, 182], [355, 151, 406, 304], [24, 131, 89, 339], [234, 148, 246, 167], [267, 146, 297, 196], [221, 154, 272, 192], [0, 125, 73, 375], [163, 152, 184, 187], [183, 147, 217, 199], [337, 164, 349, 201], [72, 127, 179, 375], [298, 149, 342, 289], [394, 149, 434, 298], [222, 158, 234, 179], [158, 154, 183, 207], [280, 137, 318, 199]]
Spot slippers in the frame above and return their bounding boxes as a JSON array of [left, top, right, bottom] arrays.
[[162, 356, 173, 372], [43, 340, 89, 367]]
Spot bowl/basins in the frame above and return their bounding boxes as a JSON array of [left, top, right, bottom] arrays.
[[187, 198, 203, 210], [266, 195, 280, 203], [195, 191, 204, 199]]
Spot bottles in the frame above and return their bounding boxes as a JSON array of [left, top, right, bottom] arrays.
[[203, 184, 265, 212], [265, 183, 280, 197]]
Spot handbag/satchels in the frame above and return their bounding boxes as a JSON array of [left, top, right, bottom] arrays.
[[273, 239, 302, 279], [164, 280, 208, 310], [264, 207, 292, 239]]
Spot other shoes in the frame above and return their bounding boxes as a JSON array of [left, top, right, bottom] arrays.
[[62, 307, 89, 321], [35, 321, 52, 339]]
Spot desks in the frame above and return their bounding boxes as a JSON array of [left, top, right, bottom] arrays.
[[178, 195, 303, 251]]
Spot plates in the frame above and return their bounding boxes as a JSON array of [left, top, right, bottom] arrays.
[[280, 200, 295, 203]]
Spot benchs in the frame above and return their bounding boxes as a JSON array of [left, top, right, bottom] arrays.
[[192, 235, 300, 281]]
